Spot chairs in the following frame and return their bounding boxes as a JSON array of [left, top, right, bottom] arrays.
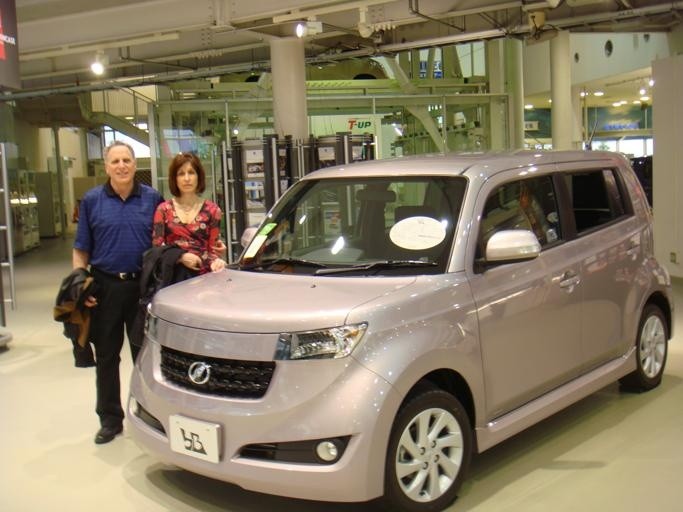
[[359, 204, 451, 269]]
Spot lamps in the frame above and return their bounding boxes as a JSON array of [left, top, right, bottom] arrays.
[[90, 49, 110, 75], [296, 19, 324, 39]]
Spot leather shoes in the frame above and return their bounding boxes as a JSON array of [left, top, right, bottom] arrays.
[[94, 418, 124, 445]]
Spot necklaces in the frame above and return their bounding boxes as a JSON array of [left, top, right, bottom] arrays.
[[176, 198, 197, 215]]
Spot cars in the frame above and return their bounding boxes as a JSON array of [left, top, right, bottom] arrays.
[[126, 150, 676, 512]]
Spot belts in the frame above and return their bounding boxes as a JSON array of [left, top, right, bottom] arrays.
[[88, 265, 141, 282]]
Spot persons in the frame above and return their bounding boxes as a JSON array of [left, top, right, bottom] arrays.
[[149, 150, 229, 290], [67, 141, 230, 446]]
